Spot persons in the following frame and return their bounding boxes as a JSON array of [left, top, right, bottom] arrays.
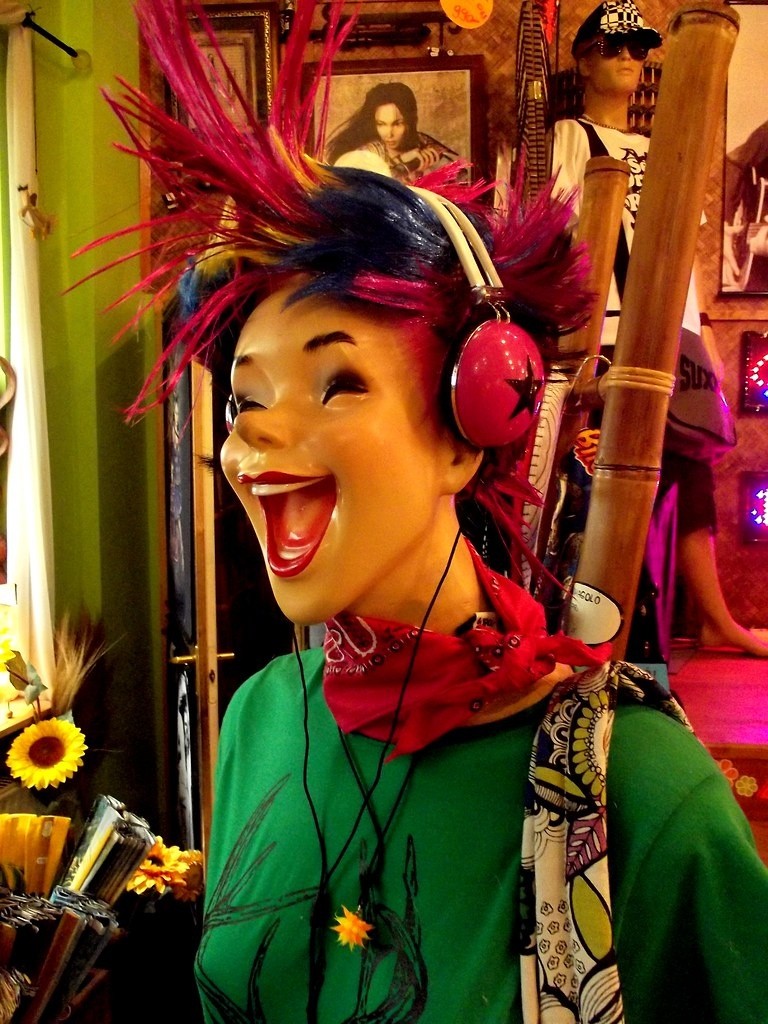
[[324, 80, 440, 187], [722, 120, 768, 294], [190, 197, 768, 1024], [549, 2, 767, 659]]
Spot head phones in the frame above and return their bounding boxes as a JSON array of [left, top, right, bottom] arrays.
[[413, 186, 544, 453]]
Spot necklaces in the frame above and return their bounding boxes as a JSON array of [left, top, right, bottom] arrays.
[[327, 722, 426, 956], [579, 115, 630, 134]]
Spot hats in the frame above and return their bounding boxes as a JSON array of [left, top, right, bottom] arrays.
[[572, 1, 665, 55]]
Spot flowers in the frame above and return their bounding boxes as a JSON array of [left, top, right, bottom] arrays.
[[330, 899, 373, 949], [7, 655, 203, 916]]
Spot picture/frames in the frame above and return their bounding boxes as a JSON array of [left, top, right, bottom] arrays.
[[169, 10, 278, 144], [718, 0, 768, 298], [300, 54, 487, 206], [740, 330, 768, 411], [739, 470, 768, 543]]
